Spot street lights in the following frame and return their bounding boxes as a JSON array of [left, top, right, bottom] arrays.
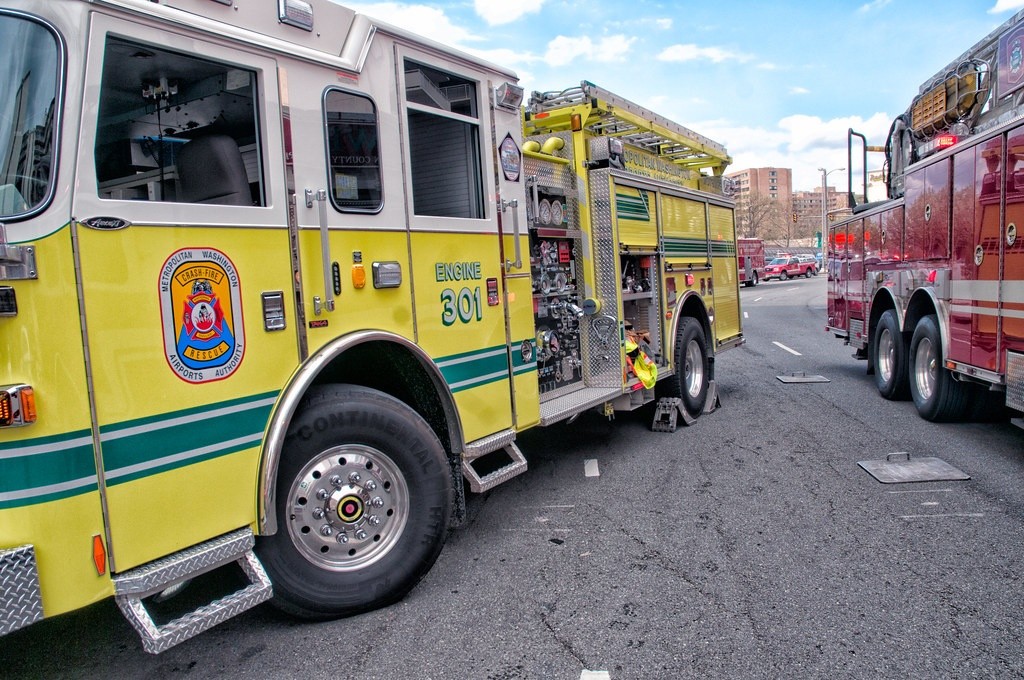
[[818, 167, 845, 272]]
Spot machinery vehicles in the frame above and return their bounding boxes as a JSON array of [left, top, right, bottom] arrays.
[[0, 0, 748, 654]]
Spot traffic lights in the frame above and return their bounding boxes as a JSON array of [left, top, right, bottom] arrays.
[[793, 213, 797, 223], [829, 209, 836, 221]]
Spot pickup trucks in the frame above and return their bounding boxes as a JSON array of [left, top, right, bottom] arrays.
[[763, 256, 815, 282]]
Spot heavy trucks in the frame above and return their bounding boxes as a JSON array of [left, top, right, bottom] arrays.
[[825, 10, 1024, 431]]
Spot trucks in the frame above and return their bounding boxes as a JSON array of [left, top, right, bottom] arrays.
[[737, 238, 765, 287]]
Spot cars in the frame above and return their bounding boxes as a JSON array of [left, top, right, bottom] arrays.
[[795, 254, 822, 272], [816, 253, 827, 268], [764, 257, 774, 266]]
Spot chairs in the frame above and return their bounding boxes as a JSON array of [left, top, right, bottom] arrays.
[[176, 135, 253, 206]]
